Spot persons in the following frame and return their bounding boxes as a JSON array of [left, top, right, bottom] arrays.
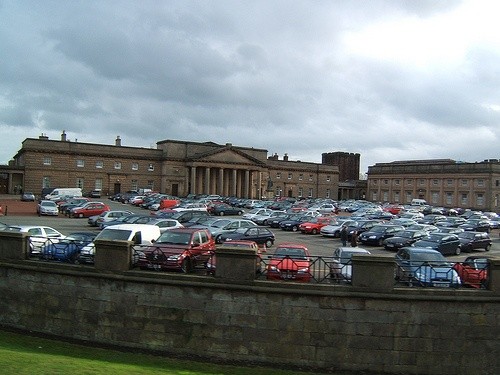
[[351, 231, 359, 246], [340, 225, 348, 245], [0, 205, 7, 216]]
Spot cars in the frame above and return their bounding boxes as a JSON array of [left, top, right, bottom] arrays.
[[0, 184, 500, 294]]
